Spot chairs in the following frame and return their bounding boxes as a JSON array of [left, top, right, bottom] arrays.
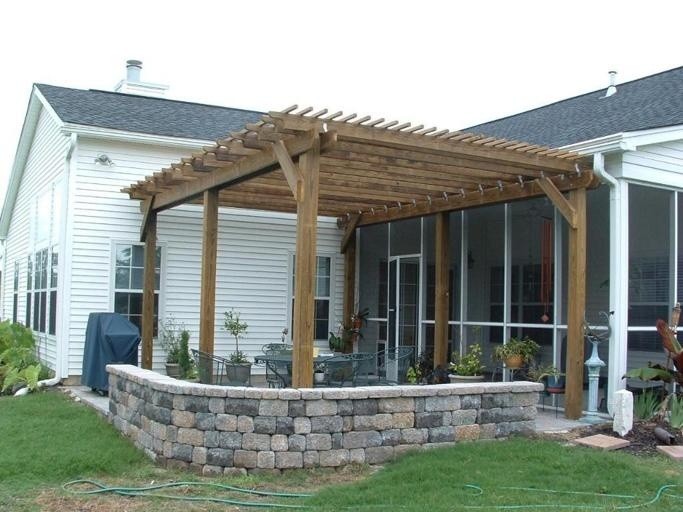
[[192, 349, 254, 388], [262, 342, 413, 387]]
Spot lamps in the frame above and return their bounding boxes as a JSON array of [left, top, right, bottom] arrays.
[[96, 155, 115, 167]]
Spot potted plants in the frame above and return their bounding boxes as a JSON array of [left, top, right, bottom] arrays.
[[220, 307, 252, 382], [448, 344, 486, 383], [329, 308, 369, 353], [158, 313, 189, 377], [177, 330, 200, 383], [491, 337, 543, 368], [536, 366, 565, 388]]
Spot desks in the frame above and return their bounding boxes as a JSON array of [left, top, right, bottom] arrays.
[[255, 356, 331, 387], [543, 388, 565, 418]]
